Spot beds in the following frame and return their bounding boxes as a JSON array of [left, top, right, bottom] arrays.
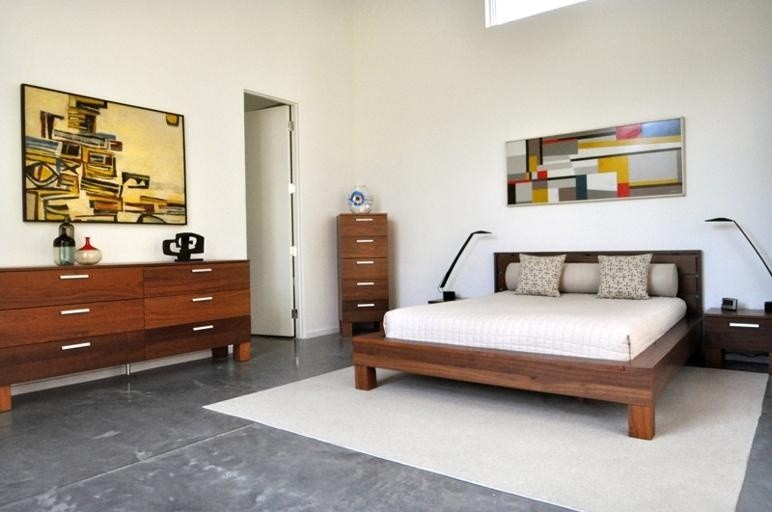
[[352, 250, 702, 440]]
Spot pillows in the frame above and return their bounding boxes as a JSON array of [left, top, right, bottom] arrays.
[[505, 254, 677, 302]]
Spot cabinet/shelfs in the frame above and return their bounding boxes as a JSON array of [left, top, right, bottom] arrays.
[[0, 260, 251, 413], [338, 214, 388, 336]]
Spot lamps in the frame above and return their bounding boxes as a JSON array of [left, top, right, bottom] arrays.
[[705, 216, 771, 314], [437, 229, 493, 299]]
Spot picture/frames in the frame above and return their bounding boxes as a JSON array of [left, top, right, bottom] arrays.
[[503, 117, 686, 207], [20, 83, 187, 223]]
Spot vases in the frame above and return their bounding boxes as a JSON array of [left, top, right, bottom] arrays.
[[53, 213, 101, 266], [349, 184, 373, 214]]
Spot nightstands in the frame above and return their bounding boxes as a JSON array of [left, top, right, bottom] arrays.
[[703, 307, 771, 374]]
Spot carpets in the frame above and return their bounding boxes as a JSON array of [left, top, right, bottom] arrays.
[[204, 365, 770, 511]]
